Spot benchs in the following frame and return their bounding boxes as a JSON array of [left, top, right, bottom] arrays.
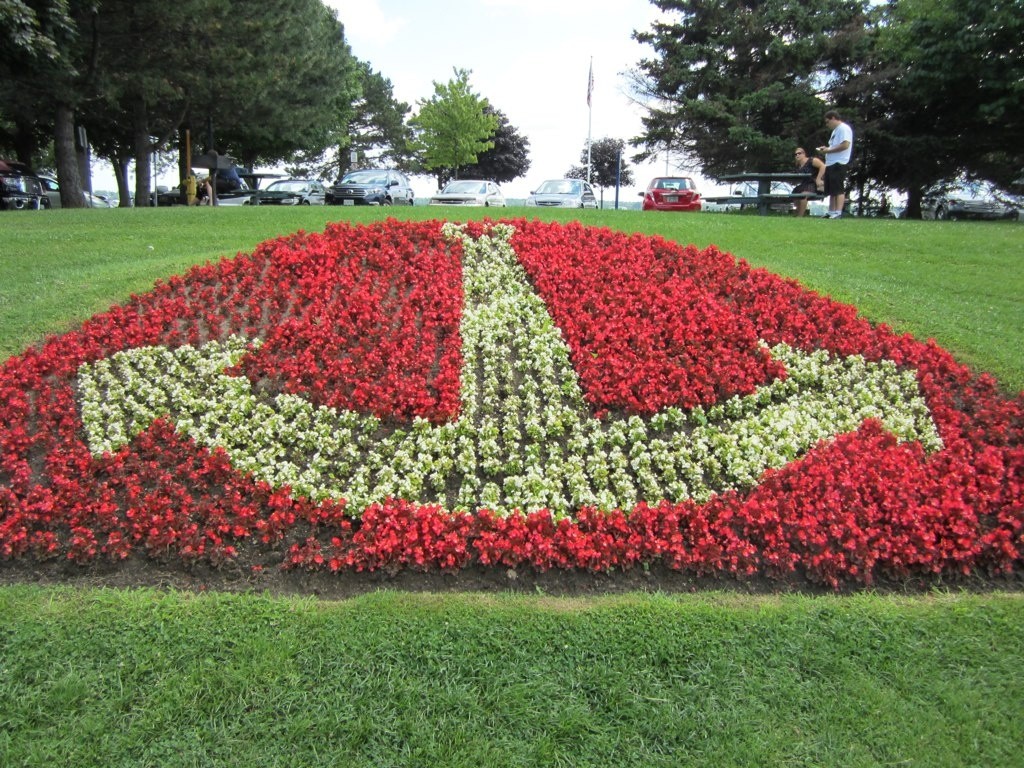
[[935, 204, 1020, 223], [702, 193, 823, 216]]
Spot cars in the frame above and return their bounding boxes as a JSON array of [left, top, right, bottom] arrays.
[[920, 187, 1024, 221], [428, 179, 507, 208], [724, 181, 810, 216], [0, 170, 327, 207], [525, 179, 599, 210], [638, 176, 702, 211]]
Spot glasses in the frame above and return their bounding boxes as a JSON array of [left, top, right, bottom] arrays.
[[794, 151, 804, 156]]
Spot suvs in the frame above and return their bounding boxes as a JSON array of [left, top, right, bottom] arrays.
[[324, 169, 415, 207]]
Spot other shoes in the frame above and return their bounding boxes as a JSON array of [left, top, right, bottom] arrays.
[[821, 212, 842, 219]]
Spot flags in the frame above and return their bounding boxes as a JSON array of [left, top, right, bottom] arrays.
[[587, 59, 594, 105]]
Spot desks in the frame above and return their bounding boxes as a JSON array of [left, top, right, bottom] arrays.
[[718, 173, 812, 216], [865, 207, 881, 217]]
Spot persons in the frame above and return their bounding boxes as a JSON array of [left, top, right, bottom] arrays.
[[790, 147, 826, 216], [819, 110, 852, 219], [197, 146, 241, 207]]
